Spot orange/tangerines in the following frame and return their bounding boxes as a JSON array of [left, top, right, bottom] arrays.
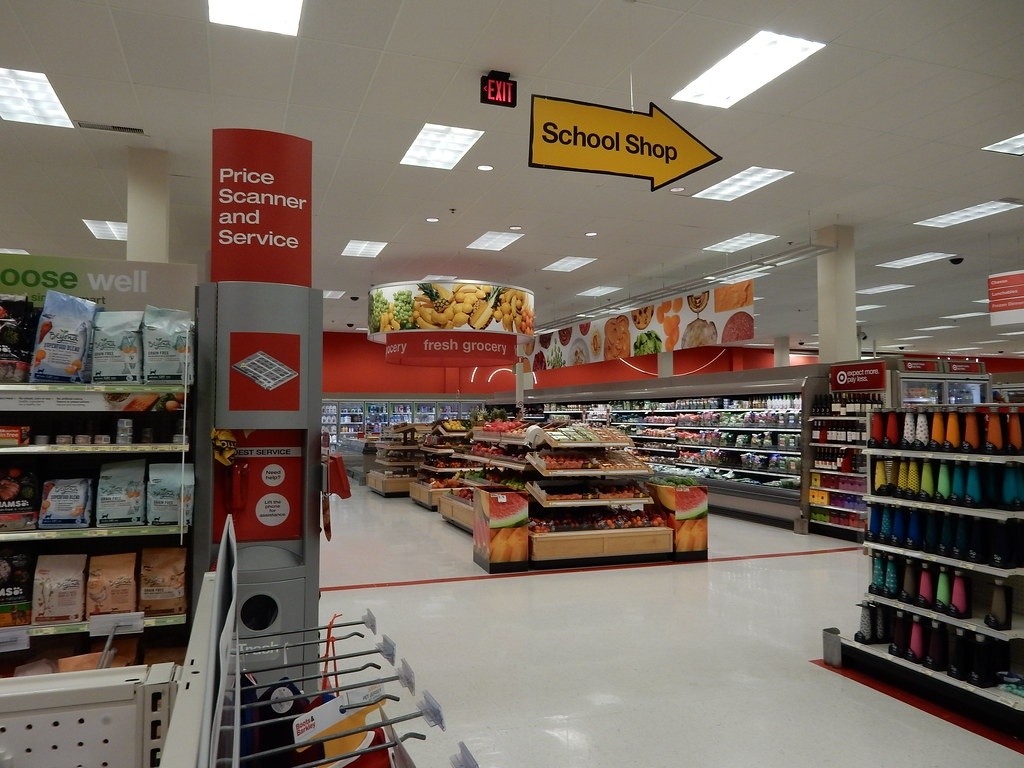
[[529, 511, 664, 532]]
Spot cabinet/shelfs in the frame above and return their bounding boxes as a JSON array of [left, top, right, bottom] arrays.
[[366, 419, 708, 574], [0, 253, 197, 636], [809, 370, 891, 544], [823, 403, 1024, 739]]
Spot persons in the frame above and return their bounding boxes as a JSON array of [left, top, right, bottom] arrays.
[[992, 391, 1006, 403], [513, 407, 524, 423]]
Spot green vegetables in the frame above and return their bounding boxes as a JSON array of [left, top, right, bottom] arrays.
[[633, 329, 662, 356]]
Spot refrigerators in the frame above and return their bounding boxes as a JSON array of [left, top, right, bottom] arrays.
[[892, 371, 994, 405]]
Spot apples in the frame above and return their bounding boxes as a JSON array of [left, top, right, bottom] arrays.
[[483, 419, 522, 432]]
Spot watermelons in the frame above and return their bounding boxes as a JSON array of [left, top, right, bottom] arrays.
[[488, 493, 529, 529], [675, 486, 708, 520]]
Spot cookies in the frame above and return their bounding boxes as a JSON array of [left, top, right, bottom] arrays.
[[631, 304, 654, 330]]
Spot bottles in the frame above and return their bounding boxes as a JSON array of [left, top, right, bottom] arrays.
[[851, 449, 867, 474], [868, 412, 978, 452], [910, 617, 924, 654], [989, 579, 1007, 625], [811, 393, 883, 416], [950, 630, 993, 675], [983, 413, 1022, 454], [859, 600, 889, 639], [917, 563, 970, 619], [1004, 463, 1024, 510], [874, 455, 983, 503], [815, 448, 845, 472], [930, 622, 944, 660], [946, 518, 1008, 568], [871, 553, 917, 603], [812, 421, 866, 445], [868, 504, 919, 549], [894, 611, 905, 659]]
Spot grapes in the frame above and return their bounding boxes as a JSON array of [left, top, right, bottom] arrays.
[[370, 288, 416, 333], [417, 283, 450, 312]]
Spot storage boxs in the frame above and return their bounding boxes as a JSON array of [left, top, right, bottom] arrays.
[[808, 473, 867, 527]]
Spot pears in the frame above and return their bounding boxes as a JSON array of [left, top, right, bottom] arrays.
[[443, 420, 466, 430]]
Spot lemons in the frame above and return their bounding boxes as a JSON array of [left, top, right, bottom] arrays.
[[431, 290, 511, 326]]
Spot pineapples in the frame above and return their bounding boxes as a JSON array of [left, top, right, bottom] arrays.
[[468, 286, 505, 330], [470, 408, 507, 426]]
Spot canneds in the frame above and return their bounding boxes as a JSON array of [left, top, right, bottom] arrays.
[[35, 418, 190, 445]]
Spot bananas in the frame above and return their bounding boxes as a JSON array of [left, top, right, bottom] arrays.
[[413, 284, 454, 329], [505, 289, 529, 332]]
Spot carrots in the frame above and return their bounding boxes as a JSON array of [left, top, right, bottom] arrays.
[[657, 298, 683, 351]]
